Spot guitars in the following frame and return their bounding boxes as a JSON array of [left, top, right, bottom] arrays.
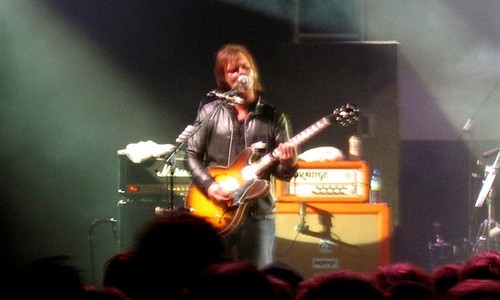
[[185, 102, 361, 241]]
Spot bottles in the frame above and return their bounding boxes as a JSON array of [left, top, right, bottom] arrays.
[[429, 222, 445, 269], [369, 168, 383, 203], [349, 135, 363, 161]]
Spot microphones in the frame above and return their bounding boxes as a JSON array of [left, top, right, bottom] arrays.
[[238, 75, 248, 86], [210, 91, 245, 104], [295, 203, 307, 232]]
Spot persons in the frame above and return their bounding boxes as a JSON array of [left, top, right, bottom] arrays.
[[0, 210, 500, 300], [183, 45, 299, 269]]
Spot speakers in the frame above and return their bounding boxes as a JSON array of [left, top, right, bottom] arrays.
[[117, 197, 172, 255], [271, 204, 390, 282]]
